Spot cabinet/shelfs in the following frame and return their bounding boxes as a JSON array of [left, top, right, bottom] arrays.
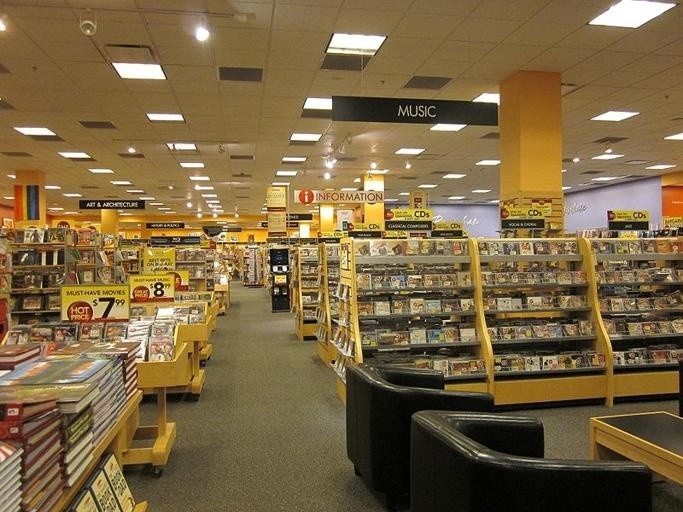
[[121, 333, 195, 475], [37, 376, 148, 512], [0, 216, 683, 411]]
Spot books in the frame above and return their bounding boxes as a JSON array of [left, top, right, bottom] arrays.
[[300, 244, 356, 375], [0, 219, 231, 364], [0, 341, 140, 512], [356, 229, 683, 379], [225, 245, 287, 294]]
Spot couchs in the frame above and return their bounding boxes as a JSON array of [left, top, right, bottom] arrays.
[[404, 407, 654, 512], [343, 361, 497, 511]]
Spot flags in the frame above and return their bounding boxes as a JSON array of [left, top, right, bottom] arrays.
[[14, 183, 40, 221]]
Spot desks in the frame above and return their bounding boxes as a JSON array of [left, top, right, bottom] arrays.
[[584, 409, 682, 486]]
